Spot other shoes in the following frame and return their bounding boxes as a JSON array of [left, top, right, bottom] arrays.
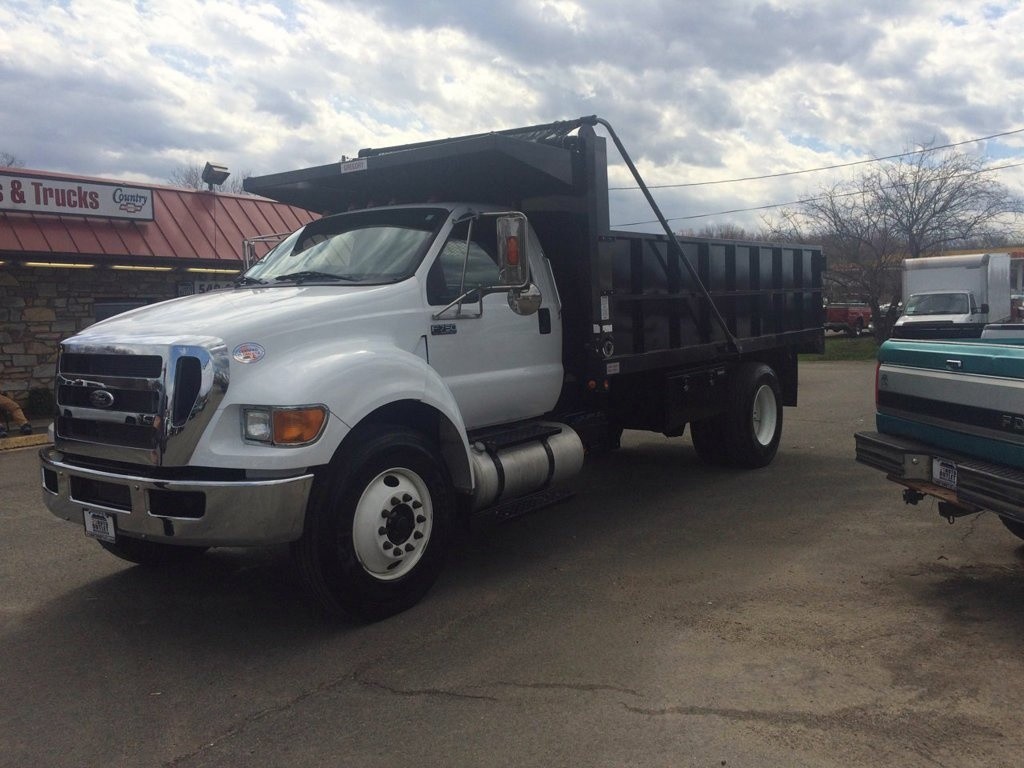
[[19, 424, 33, 435], [0, 429, 7, 438]]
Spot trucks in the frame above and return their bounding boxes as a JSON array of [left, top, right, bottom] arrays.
[[890, 252, 1014, 339]]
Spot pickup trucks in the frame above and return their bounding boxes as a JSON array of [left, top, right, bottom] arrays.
[[852, 332, 1023, 542]]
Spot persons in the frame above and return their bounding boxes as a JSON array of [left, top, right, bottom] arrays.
[[0, 395, 34, 438]]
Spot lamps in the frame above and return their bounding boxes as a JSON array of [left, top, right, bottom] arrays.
[[201, 161, 230, 192]]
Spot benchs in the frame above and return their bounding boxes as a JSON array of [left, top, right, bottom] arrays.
[[0, 382, 27, 434]]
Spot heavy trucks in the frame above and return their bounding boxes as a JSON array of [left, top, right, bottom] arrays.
[[36, 114, 826, 616]]
